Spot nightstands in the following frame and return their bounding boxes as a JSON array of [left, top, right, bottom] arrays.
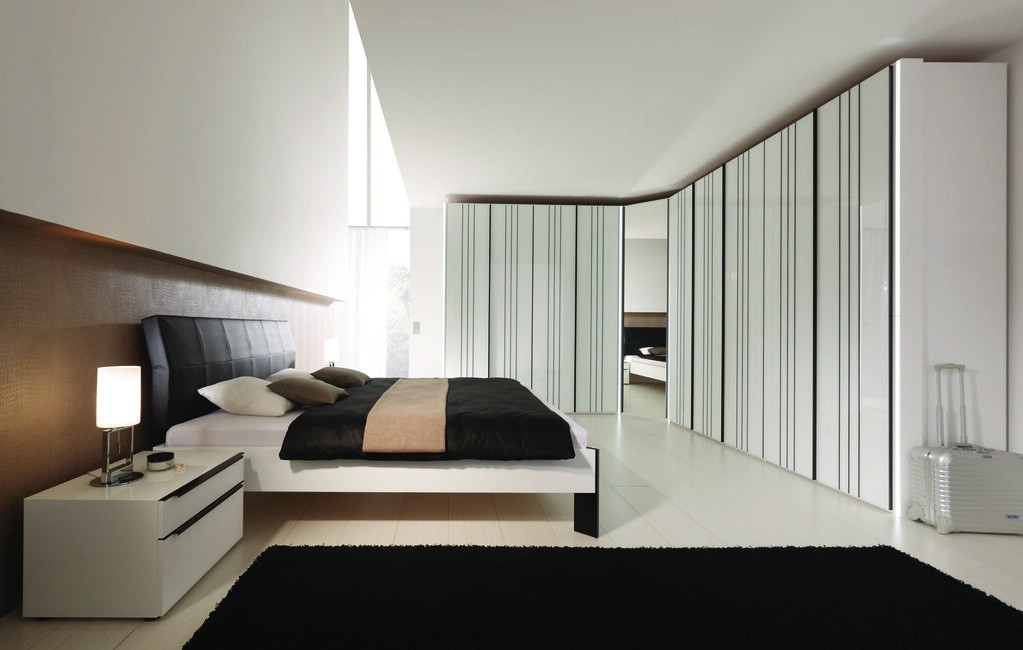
[[21, 451, 246, 620]]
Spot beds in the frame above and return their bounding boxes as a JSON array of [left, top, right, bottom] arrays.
[[625, 312, 667, 382], [142, 315, 600, 538]]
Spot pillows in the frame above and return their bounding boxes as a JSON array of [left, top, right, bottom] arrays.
[[197, 376, 295, 416], [311, 366, 373, 388], [265, 368, 311, 382], [638, 346, 654, 356], [648, 347, 665, 355], [266, 376, 350, 406]]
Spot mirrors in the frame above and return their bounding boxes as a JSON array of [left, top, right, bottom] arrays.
[[621, 199, 670, 419]]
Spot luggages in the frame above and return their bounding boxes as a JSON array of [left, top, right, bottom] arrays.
[[907, 362, 1023, 536]]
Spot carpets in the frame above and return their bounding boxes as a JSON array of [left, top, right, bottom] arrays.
[[178, 545, 1023, 650]]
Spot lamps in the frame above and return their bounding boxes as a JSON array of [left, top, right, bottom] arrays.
[[90, 366, 141, 486]]
